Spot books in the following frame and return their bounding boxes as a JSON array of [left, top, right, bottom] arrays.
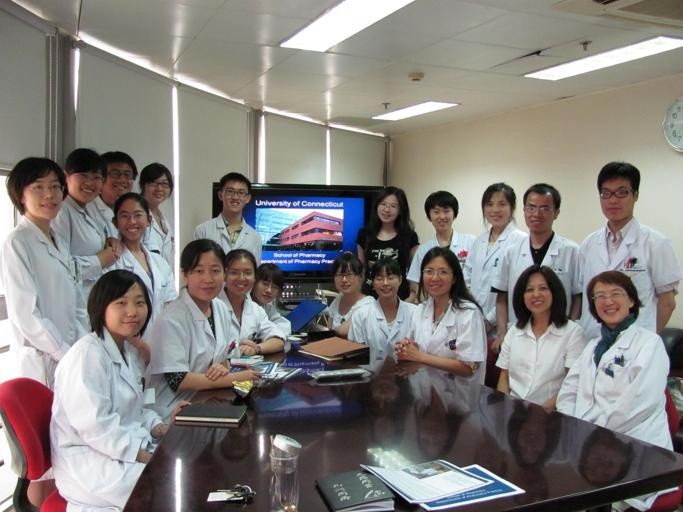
[[227, 356, 301, 384], [299, 335, 369, 363], [174, 404, 249, 428], [315, 470, 396, 512]]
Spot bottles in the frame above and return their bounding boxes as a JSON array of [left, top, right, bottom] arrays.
[[267, 434, 303, 512]]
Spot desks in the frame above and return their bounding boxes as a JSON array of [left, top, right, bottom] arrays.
[[119, 350, 683, 511]]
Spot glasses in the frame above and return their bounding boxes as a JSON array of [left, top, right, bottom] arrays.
[[147, 179, 171, 188], [422, 267, 453, 278], [600, 189, 632, 198], [24, 183, 65, 194], [591, 290, 628, 301], [106, 169, 135, 181], [334, 271, 360, 280], [222, 188, 250, 198], [523, 207, 555, 214]]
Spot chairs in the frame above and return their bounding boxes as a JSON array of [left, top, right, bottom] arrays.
[[1, 381, 66, 512], [622, 386, 683, 512], [485, 337, 503, 390]]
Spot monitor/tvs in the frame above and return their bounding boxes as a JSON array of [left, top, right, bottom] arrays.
[[213, 180, 385, 283]]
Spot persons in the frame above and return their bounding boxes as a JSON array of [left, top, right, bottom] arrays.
[[490, 183, 583, 353], [150, 238, 263, 397], [464, 182, 529, 335], [253, 264, 291, 355], [371, 355, 644, 485], [219, 250, 286, 356], [2, 150, 180, 384], [556, 269, 679, 510], [327, 254, 486, 386], [496, 265, 583, 409], [357, 187, 419, 303], [405, 191, 475, 303], [579, 162, 681, 335], [194, 172, 261, 271], [50, 268, 191, 512]]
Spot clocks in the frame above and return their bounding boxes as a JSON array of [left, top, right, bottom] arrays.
[[662, 99, 683, 153]]
